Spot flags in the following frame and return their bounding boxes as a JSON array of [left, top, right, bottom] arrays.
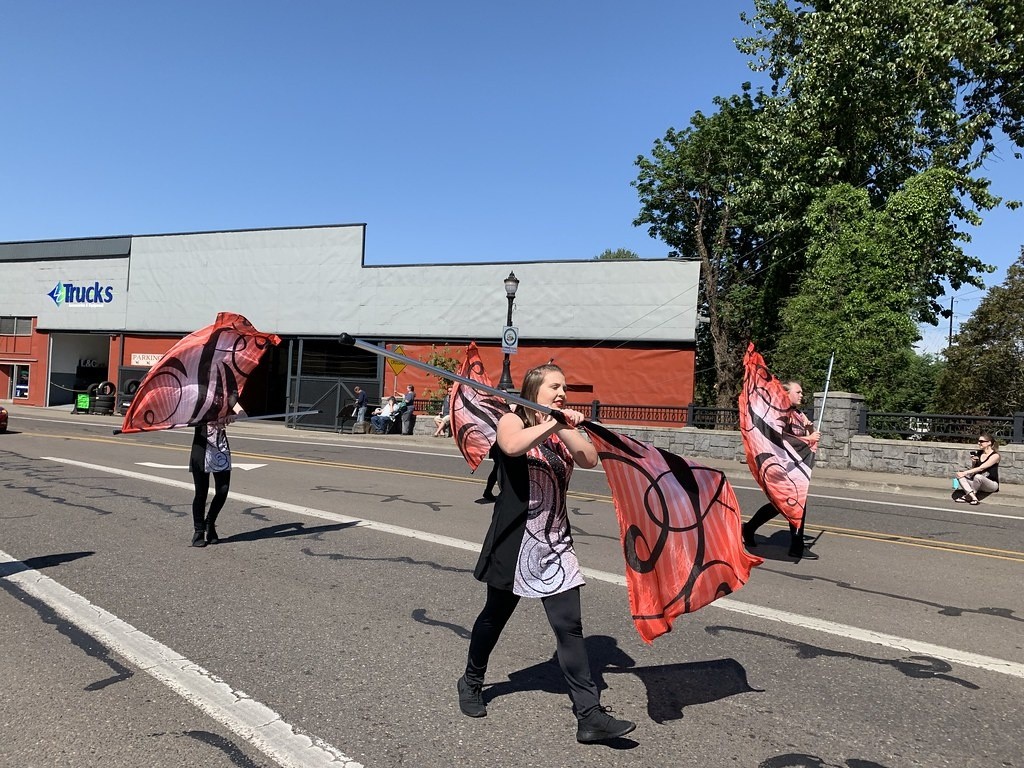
[[739, 341, 818, 529], [564, 412, 765, 646], [449, 341, 512, 471], [120, 311, 281, 432]]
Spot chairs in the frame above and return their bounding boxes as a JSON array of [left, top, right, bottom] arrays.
[[438, 413, 452, 437], [368, 403, 401, 435]]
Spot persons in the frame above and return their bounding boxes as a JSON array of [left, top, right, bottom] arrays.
[[434, 385, 453, 437], [457, 364, 637, 743], [955, 434, 1001, 505], [189, 360, 249, 547], [741, 380, 823, 559], [371, 384, 416, 435], [482, 384, 509, 502], [354, 385, 368, 422]]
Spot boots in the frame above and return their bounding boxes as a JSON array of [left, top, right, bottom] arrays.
[[457, 651, 488, 718], [576, 702, 636, 741]]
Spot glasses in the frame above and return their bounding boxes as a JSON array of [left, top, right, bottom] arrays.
[[979, 440, 989, 443]]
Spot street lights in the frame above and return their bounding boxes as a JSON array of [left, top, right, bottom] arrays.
[[496, 270, 520, 389]]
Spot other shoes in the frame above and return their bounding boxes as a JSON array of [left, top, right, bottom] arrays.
[[377, 432, 385, 435], [789, 542, 819, 560], [204, 520, 220, 544], [431, 433, 438, 437], [483, 490, 498, 501], [373, 431, 377, 434], [741, 523, 756, 548], [191, 526, 206, 547]]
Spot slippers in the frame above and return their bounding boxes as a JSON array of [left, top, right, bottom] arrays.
[[955, 498, 970, 503], [970, 500, 980, 505]]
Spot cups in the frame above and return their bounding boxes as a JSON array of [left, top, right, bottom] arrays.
[[952, 479, 959, 489]]
